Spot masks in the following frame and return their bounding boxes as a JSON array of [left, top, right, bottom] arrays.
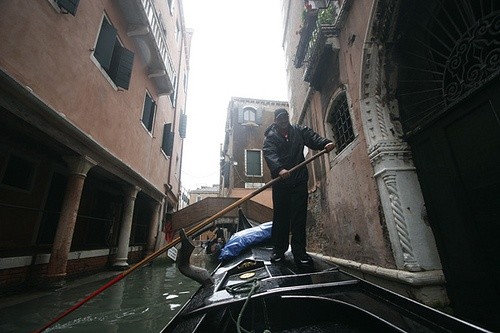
[[276, 121, 289, 128]]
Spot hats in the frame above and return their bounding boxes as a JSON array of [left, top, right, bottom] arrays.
[[274, 107, 289, 118]]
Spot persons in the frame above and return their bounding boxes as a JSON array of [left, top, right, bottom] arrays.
[[215, 227, 223, 238], [262, 107, 334, 263]]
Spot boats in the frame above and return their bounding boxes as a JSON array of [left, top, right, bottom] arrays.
[[203, 206, 253, 266]]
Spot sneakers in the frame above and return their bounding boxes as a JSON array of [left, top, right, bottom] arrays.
[[294, 253, 313, 263], [271, 251, 282, 261]]
